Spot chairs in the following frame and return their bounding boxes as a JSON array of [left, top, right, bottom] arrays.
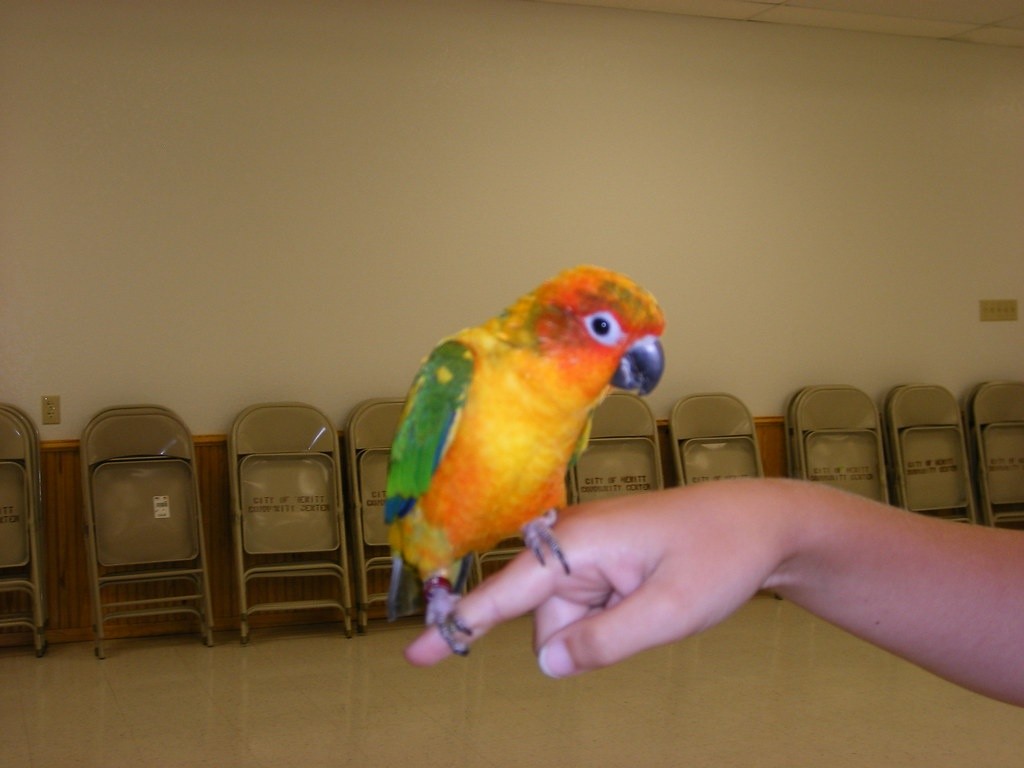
[[0, 407, 52, 657], [227, 401, 354, 645], [569, 390, 664, 505], [782, 384, 889, 506], [79, 402, 215, 660], [880, 383, 976, 525], [963, 379, 1024, 530], [471, 533, 528, 593], [343, 396, 467, 632], [668, 391, 765, 488]]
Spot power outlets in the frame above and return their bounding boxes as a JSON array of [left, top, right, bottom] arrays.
[[41, 394, 60, 425], [979, 300, 1018, 322]]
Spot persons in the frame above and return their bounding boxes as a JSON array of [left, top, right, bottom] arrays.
[[403, 477, 1024, 709]]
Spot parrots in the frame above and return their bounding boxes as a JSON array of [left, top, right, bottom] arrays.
[[381, 262, 667, 657]]
